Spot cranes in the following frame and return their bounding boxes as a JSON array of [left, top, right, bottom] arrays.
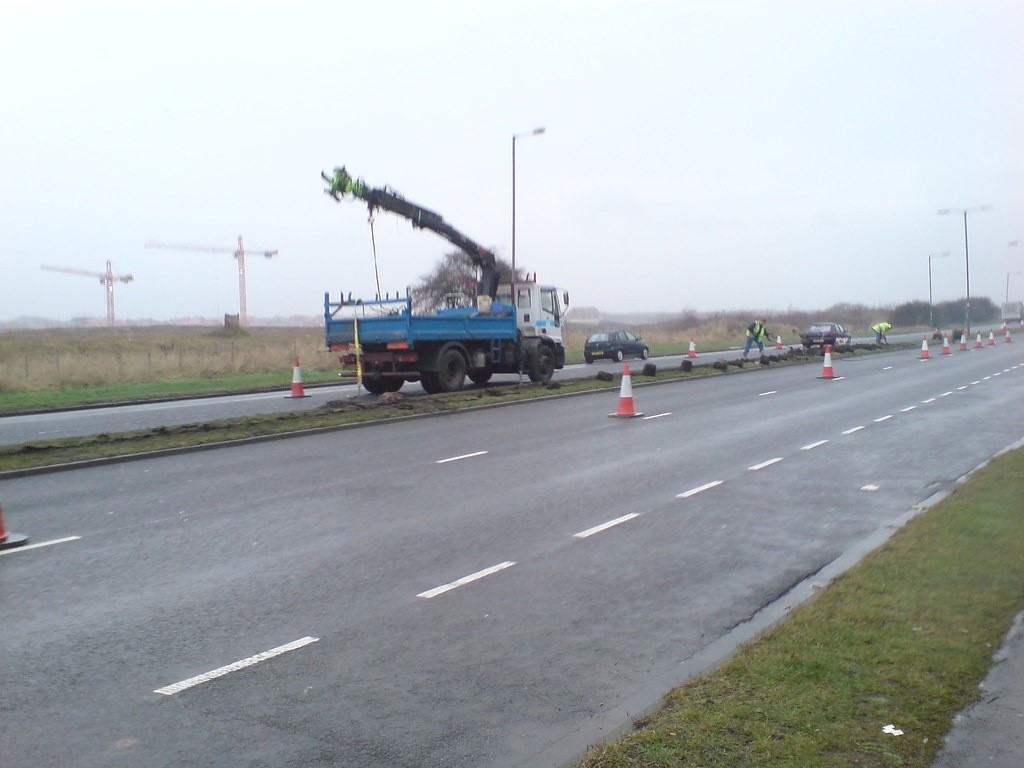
[[144, 233, 278, 327], [38, 260, 136, 328]]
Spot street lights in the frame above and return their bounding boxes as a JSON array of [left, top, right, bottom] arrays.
[[511, 127, 548, 284]]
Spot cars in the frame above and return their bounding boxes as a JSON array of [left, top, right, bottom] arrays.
[[800, 322, 852, 349], [584, 329, 649, 365]]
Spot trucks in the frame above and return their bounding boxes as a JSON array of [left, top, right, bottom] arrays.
[[323, 281, 569, 393]]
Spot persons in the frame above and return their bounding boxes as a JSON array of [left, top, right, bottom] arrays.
[[742, 319, 772, 357], [872, 322, 892, 342]]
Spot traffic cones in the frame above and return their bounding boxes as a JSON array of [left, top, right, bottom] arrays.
[[938, 332, 954, 354], [957, 330, 968, 351], [0, 496, 29, 550], [974, 329, 985, 348], [988, 330, 996, 346], [816, 344, 840, 379], [775, 332, 784, 349], [1003, 328, 1013, 343], [284, 358, 311, 398], [918, 334, 933, 359], [1000, 322, 1007, 336], [684, 336, 699, 359], [1020, 319, 1024, 328], [608, 360, 645, 418]]
